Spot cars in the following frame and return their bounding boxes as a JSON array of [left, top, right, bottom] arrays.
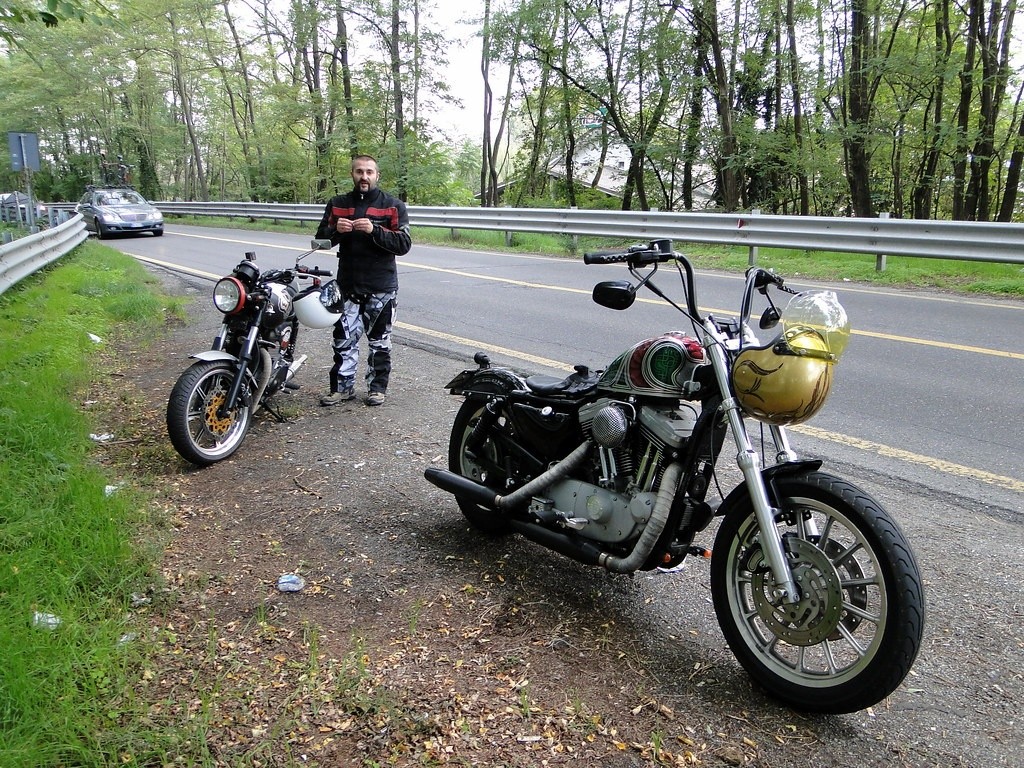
[[73, 188, 163, 241]]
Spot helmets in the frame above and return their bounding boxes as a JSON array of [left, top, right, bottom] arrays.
[[733, 325, 836, 426], [292, 281, 345, 329]]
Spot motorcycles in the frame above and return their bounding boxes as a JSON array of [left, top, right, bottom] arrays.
[[165, 238, 334, 467], [423, 238, 926, 719]]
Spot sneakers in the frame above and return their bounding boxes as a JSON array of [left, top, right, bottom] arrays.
[[320, 385, 356, 405], [366, 390, 384, 406]]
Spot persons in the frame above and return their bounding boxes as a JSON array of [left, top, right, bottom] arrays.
[[315, 155, 413, 405], [99, 196, 129, 205]]
